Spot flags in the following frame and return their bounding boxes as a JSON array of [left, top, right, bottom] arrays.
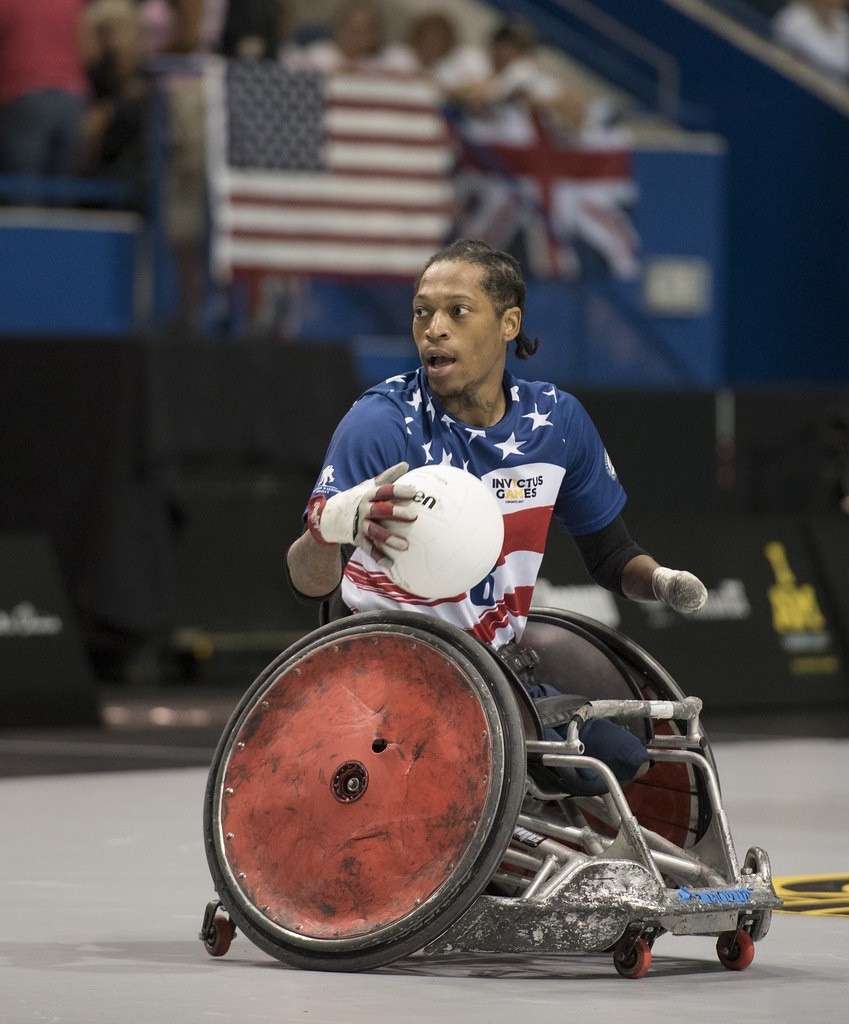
[[464, 110, 723, 389], [208, 51, 453, 276]]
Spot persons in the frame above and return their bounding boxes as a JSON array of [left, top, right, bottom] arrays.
[[228, 0, 574, 106], [764, 1, 847, 87], [0, 0, 232, 197], [283, 237, 708, 798]]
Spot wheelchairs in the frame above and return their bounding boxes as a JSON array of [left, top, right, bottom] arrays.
[[199, 584, 774, 978]]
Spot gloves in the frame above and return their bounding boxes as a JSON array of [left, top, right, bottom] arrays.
[[307, 461, 418, 571]]
